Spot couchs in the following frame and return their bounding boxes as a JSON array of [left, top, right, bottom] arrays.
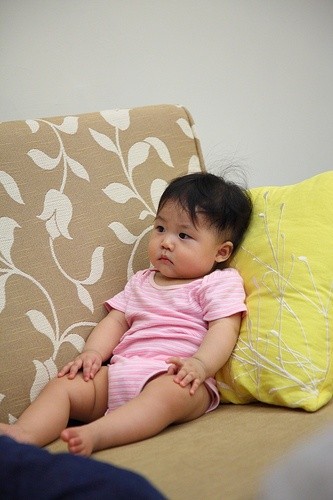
[[0, 104, 333, 500]]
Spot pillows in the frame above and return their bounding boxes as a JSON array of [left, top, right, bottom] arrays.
[[218, 170, 333, 414]]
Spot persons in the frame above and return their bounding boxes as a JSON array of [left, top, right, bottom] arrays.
[[0, 171, 254, 459]]
